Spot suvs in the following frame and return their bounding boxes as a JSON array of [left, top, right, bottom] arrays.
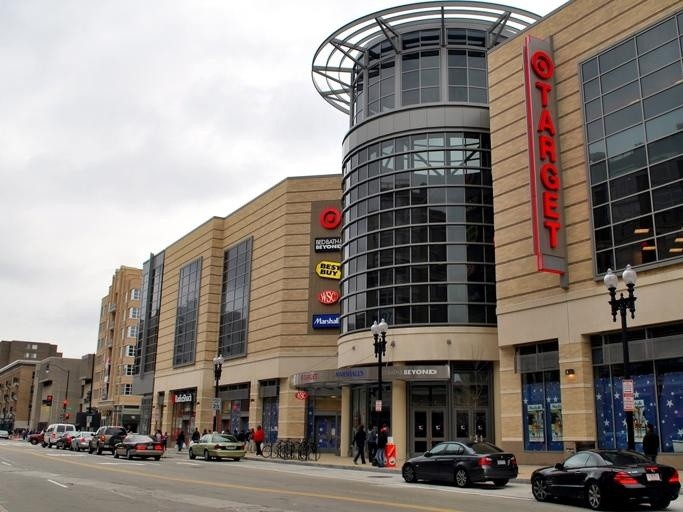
[[86, 425, 127, 455]]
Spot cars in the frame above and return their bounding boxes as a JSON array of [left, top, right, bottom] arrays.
[[0, 430, 9, 438], [55, 430, 79, 450], [113, 434, 164, 460], [68, 432, 93, 451], [26, 429, 44, 444], [530, 449, 681, 511], [401, 440, 518, 488], [187, 432, 246, 461]]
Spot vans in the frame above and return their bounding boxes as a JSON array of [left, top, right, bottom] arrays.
[[42, 423, 76, 448]]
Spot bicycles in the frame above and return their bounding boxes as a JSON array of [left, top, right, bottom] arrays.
[[260, 437, 320, 461]]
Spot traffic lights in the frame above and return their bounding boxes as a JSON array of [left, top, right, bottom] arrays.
[[62, 399, 67, 409], [65, 412, 68, 418], [46, 395, 53, 406]]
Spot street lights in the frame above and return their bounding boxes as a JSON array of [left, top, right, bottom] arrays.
[[367, 317, 388, 452], [209, 353, 224, 432], [45, 362, 69, 415], [602, 263, 636, 452]]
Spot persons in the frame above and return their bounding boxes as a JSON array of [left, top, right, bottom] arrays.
[[352, 424, 387, 468], [155, 424, 265, 456], [21, 428, 36, 441], [642, 422, 660, 463]]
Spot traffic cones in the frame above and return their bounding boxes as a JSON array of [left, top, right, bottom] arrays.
[[15, 434, 18, 439]]
